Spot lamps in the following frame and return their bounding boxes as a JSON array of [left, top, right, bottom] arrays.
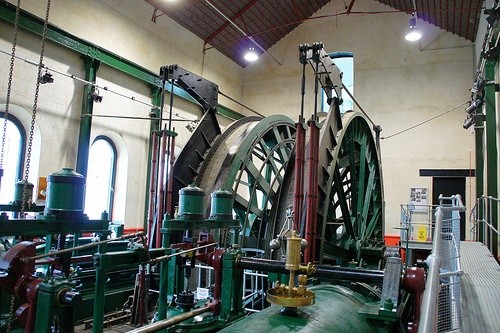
[[244, 43, 258, 62], [405, 13, 421, 42]]
